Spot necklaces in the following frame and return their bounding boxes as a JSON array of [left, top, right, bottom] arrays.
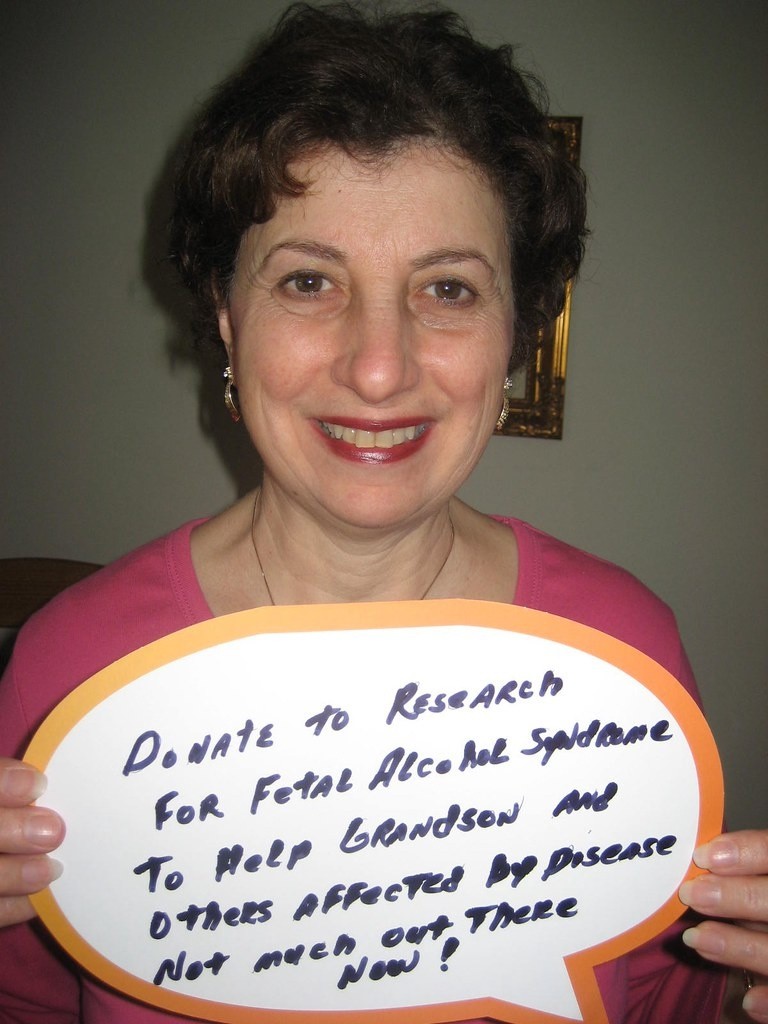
[[251, 487, 456, 607]]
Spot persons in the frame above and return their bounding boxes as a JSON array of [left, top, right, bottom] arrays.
[[0, 0, 767, 1024]]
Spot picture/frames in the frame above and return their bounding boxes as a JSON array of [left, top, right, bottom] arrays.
[[496, 115, 582, 438]]
